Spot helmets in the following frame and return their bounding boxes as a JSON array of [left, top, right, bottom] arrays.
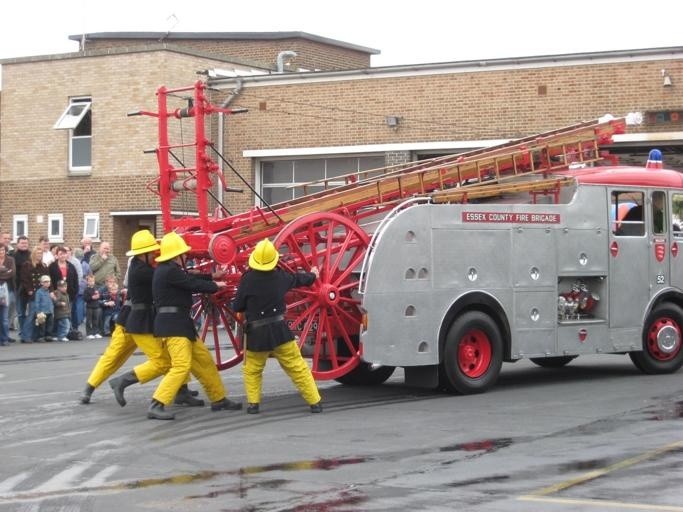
[[122, 227, 162, 258], [153, 231, 192, 263], [247, 237, 282, 272]]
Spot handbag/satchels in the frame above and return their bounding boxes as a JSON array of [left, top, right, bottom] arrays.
[[0, 282, 9, 308]]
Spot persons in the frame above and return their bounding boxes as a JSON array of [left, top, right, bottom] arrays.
[[616, 191, 680, 236]]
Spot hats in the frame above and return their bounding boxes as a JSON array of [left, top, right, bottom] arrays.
[[91, 237, 103, 243], [39, 274, 50, 283], [73, 248, 85, 258], [55, 280, 67, 287]]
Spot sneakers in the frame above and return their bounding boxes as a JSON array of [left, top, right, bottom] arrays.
[[8, 336, 16, 343], [57, 337, 69, 342], [94, 333, 103, 339], [85, 334, 94, 339], [0, 341, 9, 346], [8, 327, 17, 331], [19, 339, 33, 344], [46, 335, 57, 341], [36, 337, 47, 343], [104, 332, 113, 336]]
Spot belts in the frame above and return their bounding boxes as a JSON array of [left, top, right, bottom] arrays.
[[130, 303, 154, 312], [247, 314, 284, 328], [122, 300, 131, 306], [153, 305, 186, 316]]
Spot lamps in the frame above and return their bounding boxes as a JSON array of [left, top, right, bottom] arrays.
[[385, 115, 401, 133]]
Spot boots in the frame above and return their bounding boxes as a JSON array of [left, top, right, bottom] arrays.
[[309, 400, 322, 412], [107, 369, 141, 410], [210, 396, 243, 412], [181, 382, 201, 397], [144, 398, 175, 421], [174, 384, 204, 408], [78, 382, 96, 404], [246, 403, 259, 413]]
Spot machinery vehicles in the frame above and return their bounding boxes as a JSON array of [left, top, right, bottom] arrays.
[[265, 145, 682, 394]]
